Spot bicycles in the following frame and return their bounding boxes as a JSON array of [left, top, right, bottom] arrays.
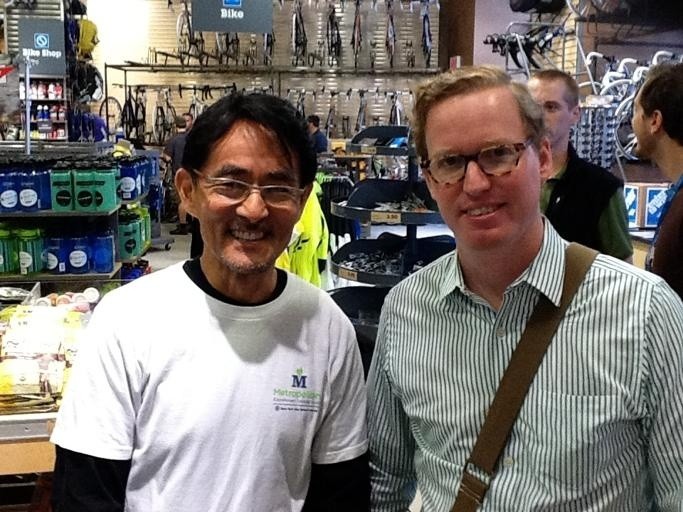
[[570, 49, 682, 165]]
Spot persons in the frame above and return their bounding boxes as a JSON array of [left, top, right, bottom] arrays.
[[162, 116, 194, 236], [307, 113, 328, 153], [362, 63, 683, 512], [525, 67, 636, 268], [42, 92, 372, 512], [166, 112, 194, 227], [622, 65, 682, 304], [81, 102, 109, 143]]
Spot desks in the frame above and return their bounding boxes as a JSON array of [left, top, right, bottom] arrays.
[[0, 305, 116, 512]]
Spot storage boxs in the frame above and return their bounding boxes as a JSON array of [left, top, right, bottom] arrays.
[[623, 181, 671, 230]]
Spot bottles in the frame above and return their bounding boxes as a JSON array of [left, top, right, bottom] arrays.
[[1, 150, 152, 306], [19, 80, 66, 120]]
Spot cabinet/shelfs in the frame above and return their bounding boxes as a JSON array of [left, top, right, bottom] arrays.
[[0, 136, 135, 292], [321, 129, 458, 364]]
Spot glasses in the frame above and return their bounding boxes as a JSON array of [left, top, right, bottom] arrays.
[[421, 138, 533, 185], [194, 169, 308, 209]]
